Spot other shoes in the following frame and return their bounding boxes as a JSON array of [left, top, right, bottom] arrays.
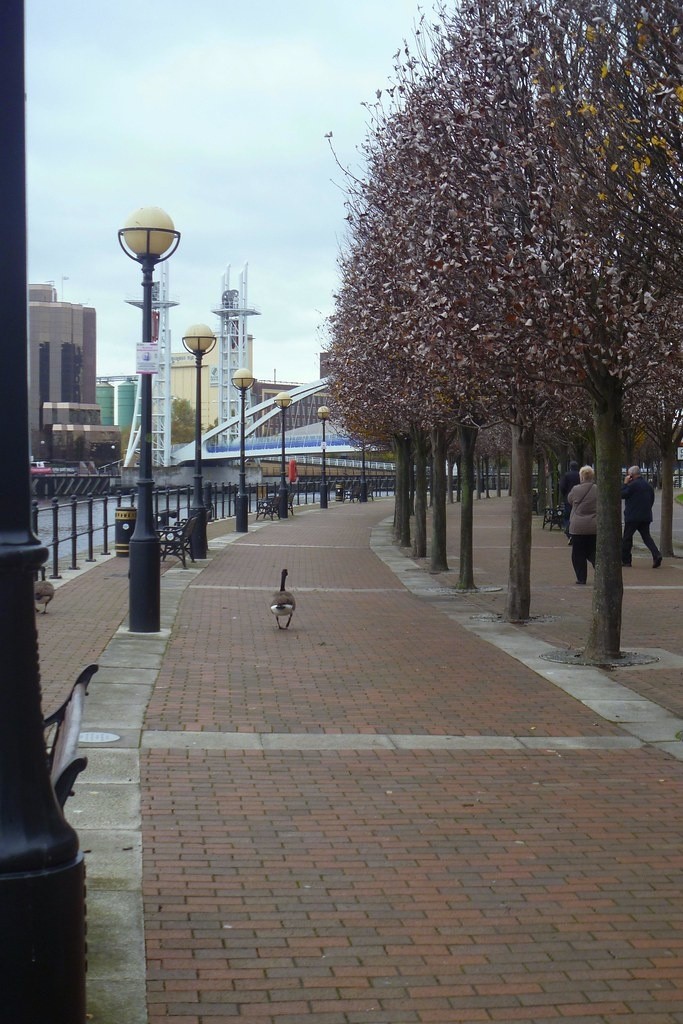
[[622, 561, 632, 566], [576, 581, 585, 584], [653, 553, 662, 568], [568, 538, 572, 545]]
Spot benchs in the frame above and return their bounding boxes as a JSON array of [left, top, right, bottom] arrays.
[[42, 662, 98, 809], [158, 506, 213, 569], [255, 488, 297, 520], [543, 503, 564, 531], [342, 482, 376, 503]]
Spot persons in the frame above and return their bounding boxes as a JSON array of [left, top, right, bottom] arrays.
[[568, 465, 597, 585], [621, 466, 663, 568], [561, 461, 579, 545]]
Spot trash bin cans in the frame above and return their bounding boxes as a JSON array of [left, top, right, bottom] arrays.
[[115, 507, 137, 556], [336, 484, 343, 501]]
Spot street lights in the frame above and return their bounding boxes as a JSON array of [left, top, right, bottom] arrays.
[[229, 367, 256, 533], [273, 391, 294, 519], [181, 321, 218, 560], [316, 405, 331, 508], [116, 205, 183, 635]]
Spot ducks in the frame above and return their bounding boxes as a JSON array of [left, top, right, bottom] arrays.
[[33, 566, 55, 614], [270, 569, 296, 629]]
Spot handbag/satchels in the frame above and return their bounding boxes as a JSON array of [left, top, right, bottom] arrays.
[[564, 525, 569, 535]]
